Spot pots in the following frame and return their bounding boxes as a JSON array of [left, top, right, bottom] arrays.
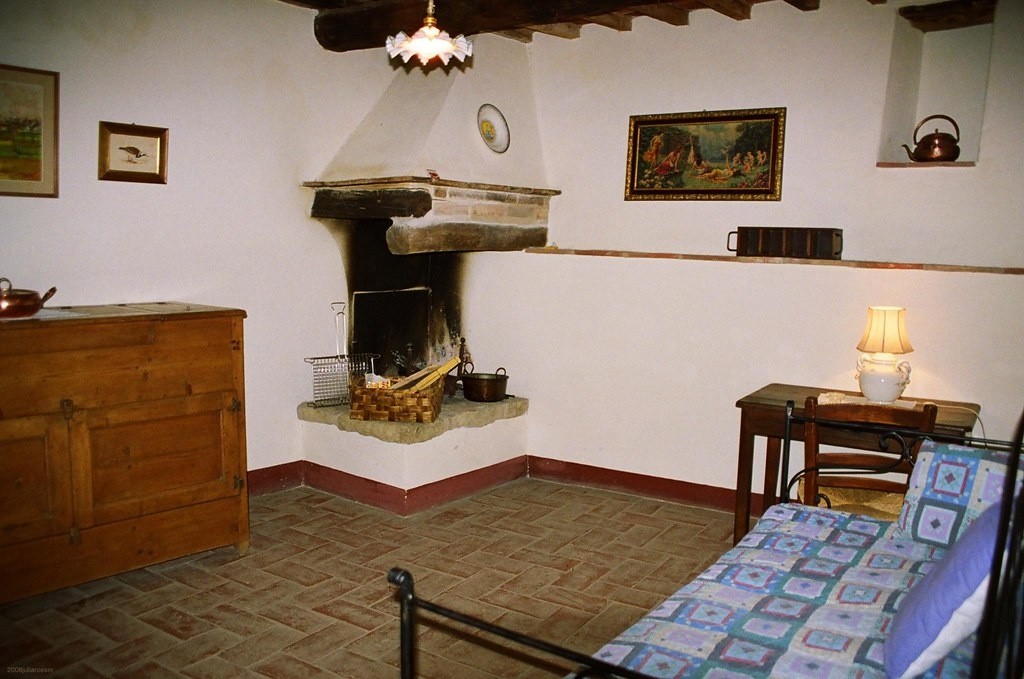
[[0, 278, 57, 318], [461, 362, 509, 402]]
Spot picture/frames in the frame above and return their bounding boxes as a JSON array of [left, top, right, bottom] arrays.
[[623, 106, 788, 201], [99, 120, 169, 184], [0, 63, 60, 198]]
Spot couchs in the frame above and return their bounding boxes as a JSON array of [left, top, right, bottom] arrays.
[[387, 400, 1024, 679]]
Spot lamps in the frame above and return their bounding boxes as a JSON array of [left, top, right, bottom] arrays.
[[385, 0, 474, 66], [855, 305, 914, 404]]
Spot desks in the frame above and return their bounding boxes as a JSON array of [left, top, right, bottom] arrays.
[[734, 383, 981, 547]]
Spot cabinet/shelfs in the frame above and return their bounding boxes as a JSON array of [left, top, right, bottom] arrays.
[[0, 301, 250, 608]]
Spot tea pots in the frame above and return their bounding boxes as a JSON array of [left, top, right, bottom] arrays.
[[902, 115, 960, 162]]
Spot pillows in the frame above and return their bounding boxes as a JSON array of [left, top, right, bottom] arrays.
[[882, 497, 1016, 679], [891, 440, 1024, 561]]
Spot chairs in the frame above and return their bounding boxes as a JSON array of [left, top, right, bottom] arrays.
[[797, 396, 939, 521]]
